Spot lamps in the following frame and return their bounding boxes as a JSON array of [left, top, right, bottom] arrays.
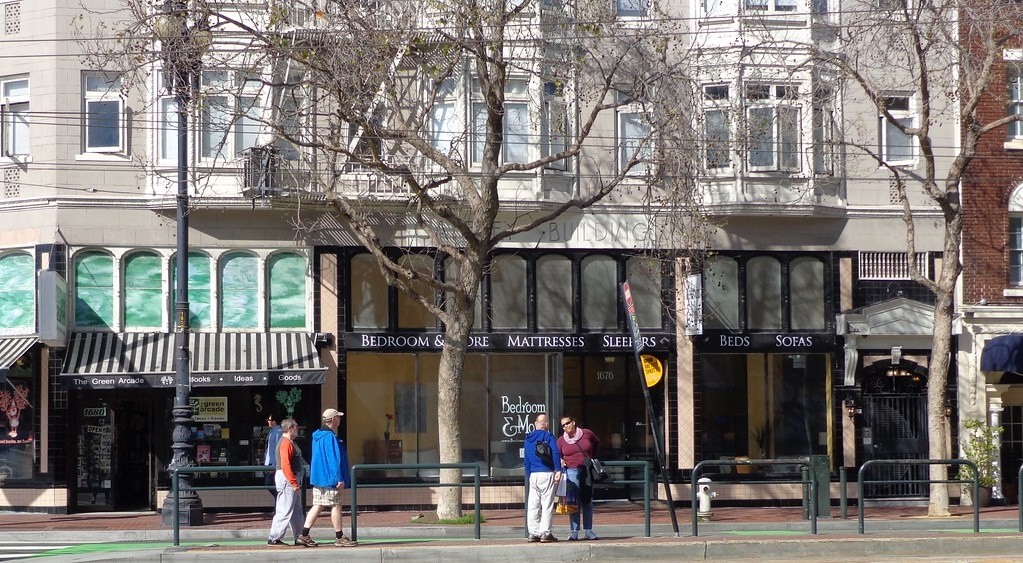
[[489, 440, 506, 468], [313, 333, 327, 346]]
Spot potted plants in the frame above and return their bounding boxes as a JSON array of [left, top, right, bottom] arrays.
[[955, 419, 1005, 508]]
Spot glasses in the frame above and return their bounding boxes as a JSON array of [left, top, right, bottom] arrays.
[[563, 421, 572, 427]]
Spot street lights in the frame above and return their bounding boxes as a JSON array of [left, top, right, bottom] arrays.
[[154, 0, 204, 527]]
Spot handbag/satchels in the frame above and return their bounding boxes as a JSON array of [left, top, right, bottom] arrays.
[[556, 482, 579, 515], [589, 459, 610, 482], [535, 432, 554, 461], [555, 466, 567, 497]]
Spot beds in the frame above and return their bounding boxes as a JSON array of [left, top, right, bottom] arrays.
[[401, 449, 491, 478]]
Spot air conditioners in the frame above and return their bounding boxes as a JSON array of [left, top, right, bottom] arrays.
[[243, 148, 276, 198]]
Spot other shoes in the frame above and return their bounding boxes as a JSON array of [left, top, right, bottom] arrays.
[[266, 514, 273, 520]]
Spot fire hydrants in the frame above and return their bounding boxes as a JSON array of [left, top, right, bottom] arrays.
[[697, 477, 718, 523]]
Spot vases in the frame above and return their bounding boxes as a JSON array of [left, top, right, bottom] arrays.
[[384, 431, 390, 440]]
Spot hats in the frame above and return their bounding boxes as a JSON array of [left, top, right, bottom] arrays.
[[322, 408, 344, 421]]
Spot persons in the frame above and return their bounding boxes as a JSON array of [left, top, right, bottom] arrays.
[[263, 414, 307, 546], [523, 414, 561, 543], [556, 417, 601, 541], [296, 409, 358, 548]]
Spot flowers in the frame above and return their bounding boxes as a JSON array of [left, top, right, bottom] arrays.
[[385, 413, 393, 433]]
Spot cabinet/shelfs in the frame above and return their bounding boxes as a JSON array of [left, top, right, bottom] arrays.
[[363, 440, 402, 479], [250, 436, 305, 486], [193, 438, 232, 482]]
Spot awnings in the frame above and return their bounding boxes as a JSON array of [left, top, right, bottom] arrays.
[[57, 330, 331, 388], [0, 336, 42, 370]]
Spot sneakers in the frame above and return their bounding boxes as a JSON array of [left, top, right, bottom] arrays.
[[528, 534, 540, 542], [268, 539, 288, 547], [539, 532, 558, 542], [334, 534, 357, 547], [569, 529, 578, 541], [296, 534, 318, 547], [584, 530, 597, 540]]
